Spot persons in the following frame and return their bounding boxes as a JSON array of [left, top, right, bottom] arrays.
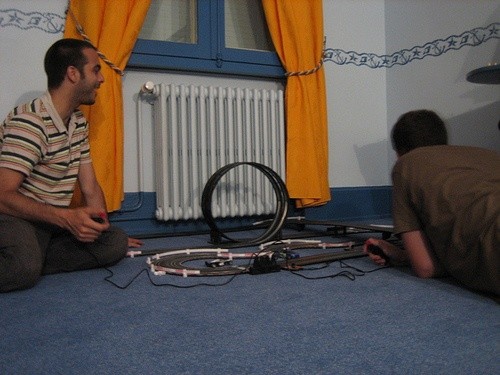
[[363, 109, 500, 284], [0, 38, 145, 293]]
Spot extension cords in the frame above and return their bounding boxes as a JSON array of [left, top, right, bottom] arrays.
[[249, 263, 281, 274]]
